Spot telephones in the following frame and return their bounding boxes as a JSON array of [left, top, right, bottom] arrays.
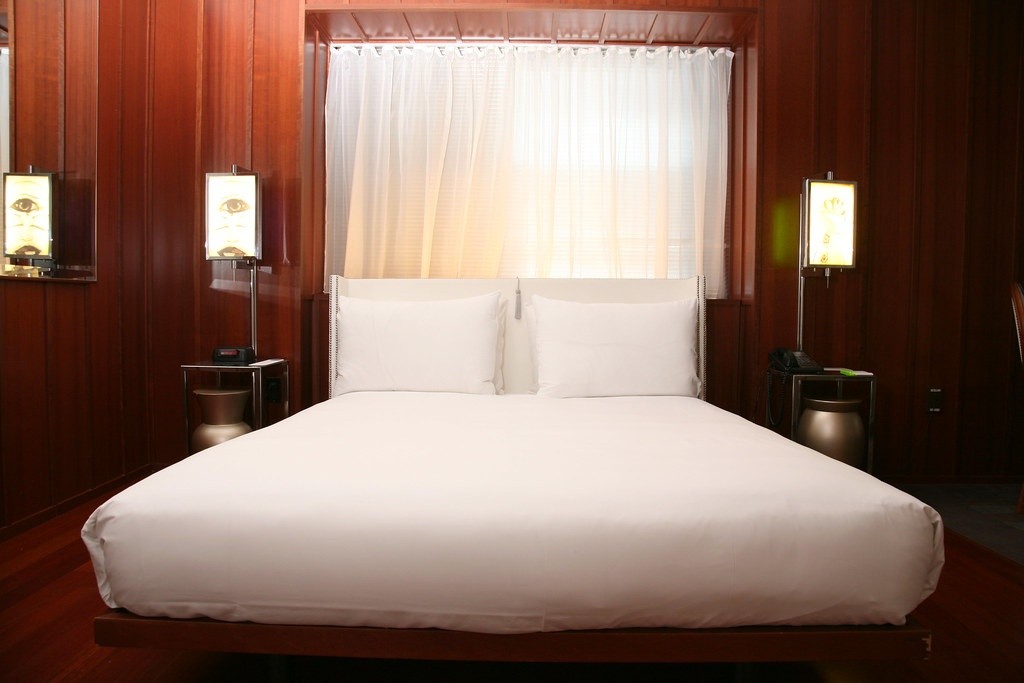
[[768, 346, 822, 373]]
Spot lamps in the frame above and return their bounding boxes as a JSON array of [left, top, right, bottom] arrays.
[[2, 162, 67, 277], [782, 170, 857, 369], [205, 164, 266, 366]]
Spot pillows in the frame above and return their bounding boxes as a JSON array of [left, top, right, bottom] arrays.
[[523, 292, 701, 401], [330, 291, 506, 394]]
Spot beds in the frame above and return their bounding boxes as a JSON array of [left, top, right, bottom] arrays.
[[67, 273, 947, 664]]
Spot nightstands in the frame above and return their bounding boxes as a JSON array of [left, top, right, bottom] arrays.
[[840, 398, 846, 401]]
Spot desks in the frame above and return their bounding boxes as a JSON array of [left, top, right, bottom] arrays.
[[177, 357, 289, 456], [765, 367, 876, 479]]
